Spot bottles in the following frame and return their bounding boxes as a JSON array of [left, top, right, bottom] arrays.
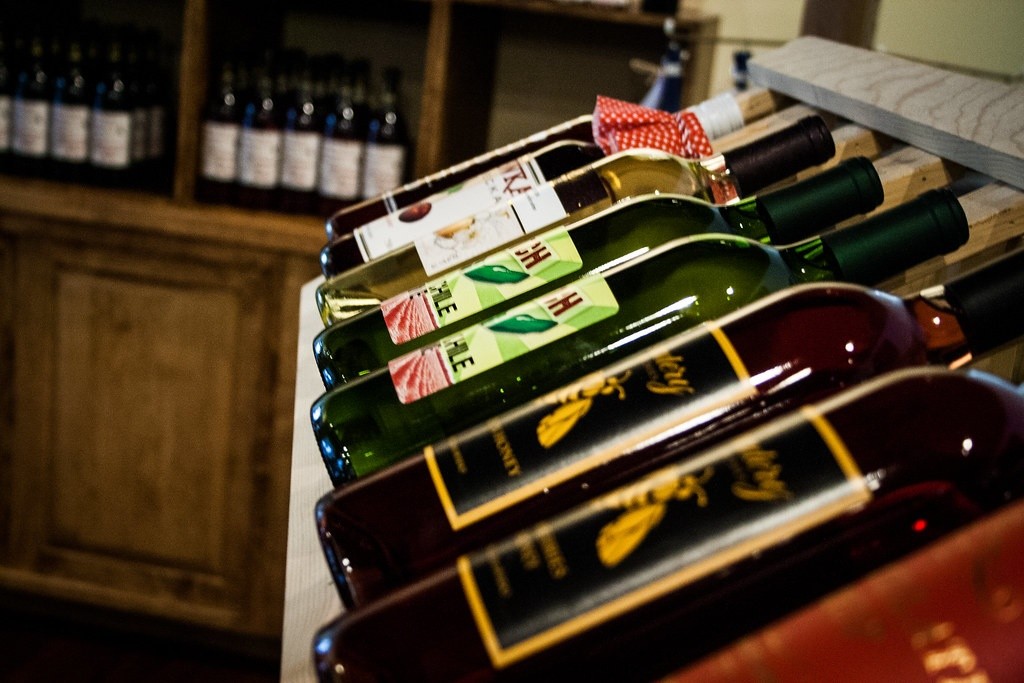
[[194, 46, 408, 215], [312, 155, 887, 393], [0, 34, 172, 183], [312, 187, 970, 482], [313, 250, 1024, 612], [323, 97, 713, 281], [325, 90, 742, 246], [309, 366, 1022, 680], [314, 114, 837, 330]]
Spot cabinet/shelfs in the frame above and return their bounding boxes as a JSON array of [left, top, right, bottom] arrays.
[[0, 0, 720, 638]]
